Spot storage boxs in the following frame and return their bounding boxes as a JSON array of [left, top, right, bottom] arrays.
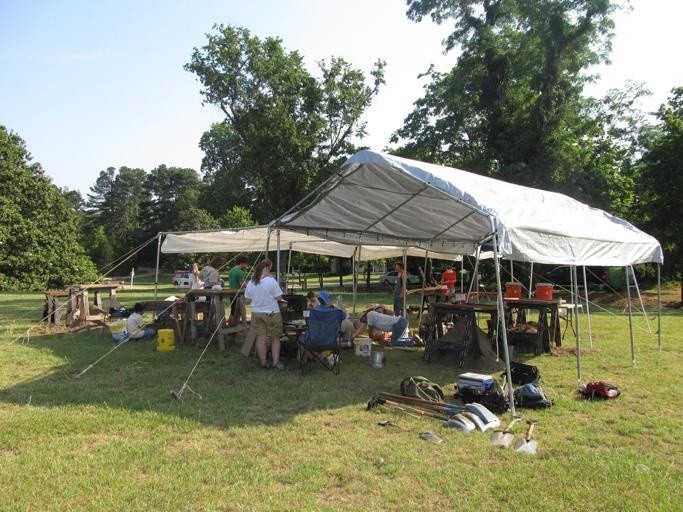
[[537, 283, 553, 299], [505, 281, 521, 297]]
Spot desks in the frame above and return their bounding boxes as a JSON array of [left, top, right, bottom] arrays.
[[43, 282, 121, 326], [182, 287, 248, 351], [417, 289, 581, 371]]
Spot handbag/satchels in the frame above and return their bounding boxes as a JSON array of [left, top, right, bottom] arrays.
[[505, 361, 539, 386]]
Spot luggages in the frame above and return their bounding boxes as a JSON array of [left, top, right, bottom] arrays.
[[454, 372, 493, 398]]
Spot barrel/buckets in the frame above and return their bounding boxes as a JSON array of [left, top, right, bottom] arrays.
[[371, 351, 385, 368], [109, 319, 128, 343], [505, 282, 521, 299], [535, 282, 554, 301], [157, 329, 176, 352]]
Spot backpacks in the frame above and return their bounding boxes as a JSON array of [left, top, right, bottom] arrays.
[[578, 380, 621, 401], [399, 376, 445, 402], [514, 383, 554, 408]]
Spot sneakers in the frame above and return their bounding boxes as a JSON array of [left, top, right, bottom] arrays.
[[260, 360, 285, 370], [413, 334, 424, 346]]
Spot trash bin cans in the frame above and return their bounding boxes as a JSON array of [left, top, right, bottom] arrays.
[[458, 269, 469, 283], [609, 267, 625, 292]]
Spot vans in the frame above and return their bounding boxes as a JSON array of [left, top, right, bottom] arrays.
[[172, 270, 196, 289], [545, 266, 600, 283]]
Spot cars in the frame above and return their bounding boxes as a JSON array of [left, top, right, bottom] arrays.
[[380, 270, 421, 285]]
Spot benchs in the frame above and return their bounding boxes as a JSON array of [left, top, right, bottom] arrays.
[[141, 299, 212, 327]]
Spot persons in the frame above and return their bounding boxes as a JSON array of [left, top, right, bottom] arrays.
[[307, 291, 353, 347], [441, 265, 457, 294], [359, 261, 425, 346], [126, 303, 157, 339], [188, 255, 285, 370]]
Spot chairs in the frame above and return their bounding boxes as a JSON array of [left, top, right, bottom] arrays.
[[285, 306, 346, 375]]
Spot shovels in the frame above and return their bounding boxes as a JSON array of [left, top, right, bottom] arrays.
[[367, 392, 501, 444], [489, 416, 540, 454]]
